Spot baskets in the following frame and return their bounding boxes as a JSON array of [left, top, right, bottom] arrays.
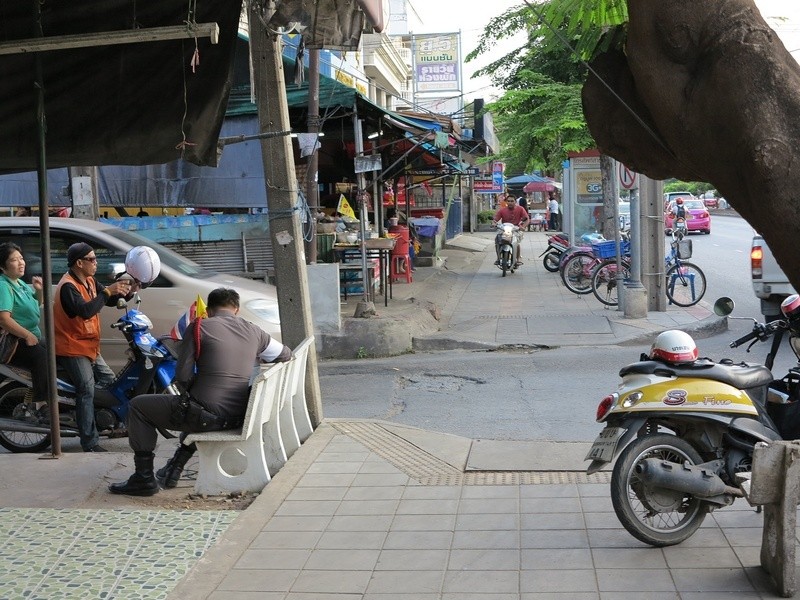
[[672, 239, 693, 259]]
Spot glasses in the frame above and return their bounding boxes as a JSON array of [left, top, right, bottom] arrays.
[[82, 257, 95, 263]]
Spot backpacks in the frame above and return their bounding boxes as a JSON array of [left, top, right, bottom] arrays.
[[677, 205, 685, 218]]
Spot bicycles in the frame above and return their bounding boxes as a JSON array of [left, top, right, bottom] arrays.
[[560, 228, 707, 307]]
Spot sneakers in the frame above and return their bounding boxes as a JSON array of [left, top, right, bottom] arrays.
[[84, 446, 108, 453], [37, 405, 52, 426]]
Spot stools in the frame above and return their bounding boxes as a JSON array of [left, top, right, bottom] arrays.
[[392, 254, 414, 283]]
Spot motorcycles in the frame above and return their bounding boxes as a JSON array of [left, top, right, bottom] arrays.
[[586, 291, 800, 548], [538, 216, 628, 273], [675, 217, 686, 240], [486, 215, 527, 277], [0, 293, 183, 452]]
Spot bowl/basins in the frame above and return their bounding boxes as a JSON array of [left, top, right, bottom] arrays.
[[345, 236, 357, 243], [390, 218, 399, 226], [344, 221, 372, 230], [317, 223, 337, 233]]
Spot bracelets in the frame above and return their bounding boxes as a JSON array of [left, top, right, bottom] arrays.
[[104, 288, 111, 296]]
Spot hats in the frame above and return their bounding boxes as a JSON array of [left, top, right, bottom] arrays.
[[66, 242, 93, 268]]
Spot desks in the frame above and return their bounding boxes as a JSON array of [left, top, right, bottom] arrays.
[[528, 223, 543, 232], [337, 240, 397, 307]]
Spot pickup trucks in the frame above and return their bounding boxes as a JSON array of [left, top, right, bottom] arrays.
[[751, 231, 800, 323]]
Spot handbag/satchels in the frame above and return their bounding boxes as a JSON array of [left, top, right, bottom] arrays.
[[0, 329, 18, 365]]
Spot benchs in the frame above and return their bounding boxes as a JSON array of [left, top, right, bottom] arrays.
[[181, 334, 316, 500], [341, 261, 376, 305]]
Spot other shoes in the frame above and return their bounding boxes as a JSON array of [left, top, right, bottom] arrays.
[[494, 260, 500, 265], [517, 261, 523, 265]]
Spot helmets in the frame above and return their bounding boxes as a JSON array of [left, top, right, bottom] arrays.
[[649, 329, 698, 364], [676, 197, 683, 204], [125, 246, 161, 283]]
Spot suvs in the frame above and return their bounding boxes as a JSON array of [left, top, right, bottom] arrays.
[[664, 192, 694, 209]]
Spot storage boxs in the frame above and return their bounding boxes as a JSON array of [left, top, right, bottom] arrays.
[[366, 237, 396, 248], [592, 239, 625, 259], [319, 222, 337, 233]]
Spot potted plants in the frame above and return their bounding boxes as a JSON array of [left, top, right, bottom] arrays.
[[477, 209, 498, 232]]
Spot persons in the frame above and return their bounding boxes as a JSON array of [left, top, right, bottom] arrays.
[[492, 195, 530, 265], [0, 242, 48, 423], [549, 196, 559, 229], [16, 207, 31, 217], [53, 242, 141, 452], [516, 194, 528, 212], [673, 198, 688, 229], [108, 288, 292, 495]]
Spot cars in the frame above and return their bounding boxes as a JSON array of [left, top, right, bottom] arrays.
[[0, 215, 283, 381], [703, 194, 718, 209], [664, 200, 710, 234]]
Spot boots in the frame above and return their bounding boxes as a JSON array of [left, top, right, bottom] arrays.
[[156, 448, 189, 487], [111, 455, 158, 496]]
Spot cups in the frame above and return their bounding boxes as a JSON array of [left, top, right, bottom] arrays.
[[383, 230, 388, 237]]
